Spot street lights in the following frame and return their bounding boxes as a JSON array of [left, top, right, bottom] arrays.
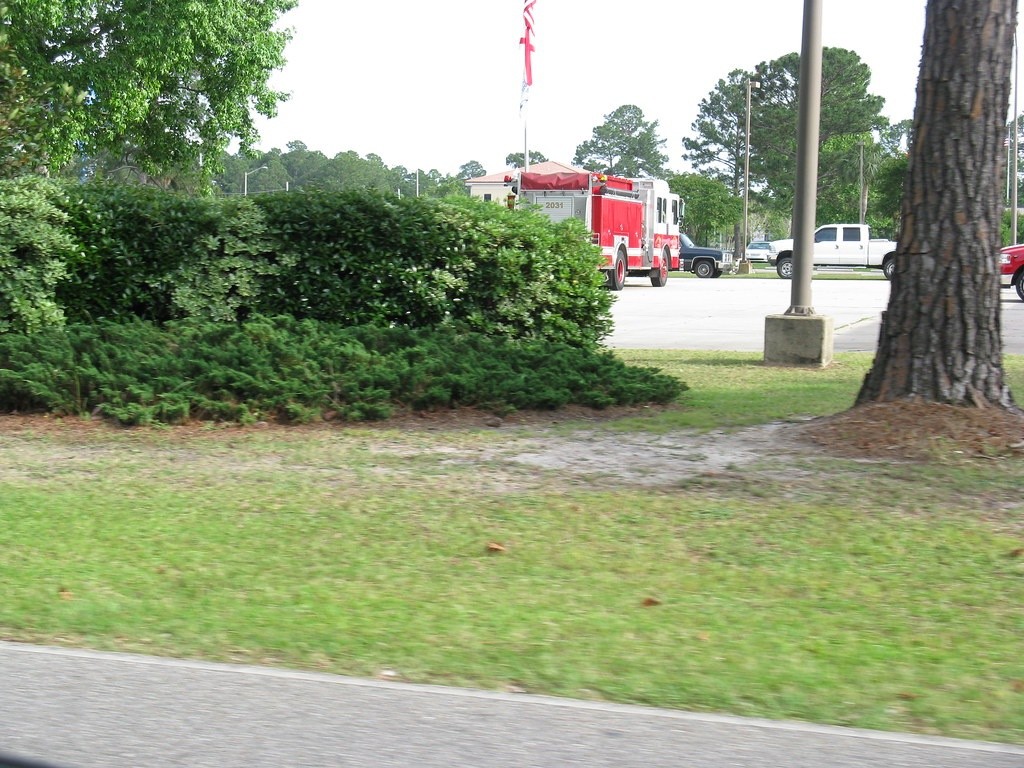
[[855, 141, 866, 224], [738, 77, 761, 274], [244, 166, 269, 197]]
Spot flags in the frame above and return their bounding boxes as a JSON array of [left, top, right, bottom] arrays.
[[519, 0, 536, 109]]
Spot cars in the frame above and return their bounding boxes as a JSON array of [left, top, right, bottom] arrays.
[[746, 241, 772, 263], [1000, 243, 1024, 303]]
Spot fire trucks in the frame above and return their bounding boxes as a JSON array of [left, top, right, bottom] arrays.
[[502, 171, 686, 291]]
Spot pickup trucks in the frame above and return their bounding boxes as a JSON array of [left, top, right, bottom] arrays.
[[678, 231, 732, 278], [767, 222, 898, 280]]
